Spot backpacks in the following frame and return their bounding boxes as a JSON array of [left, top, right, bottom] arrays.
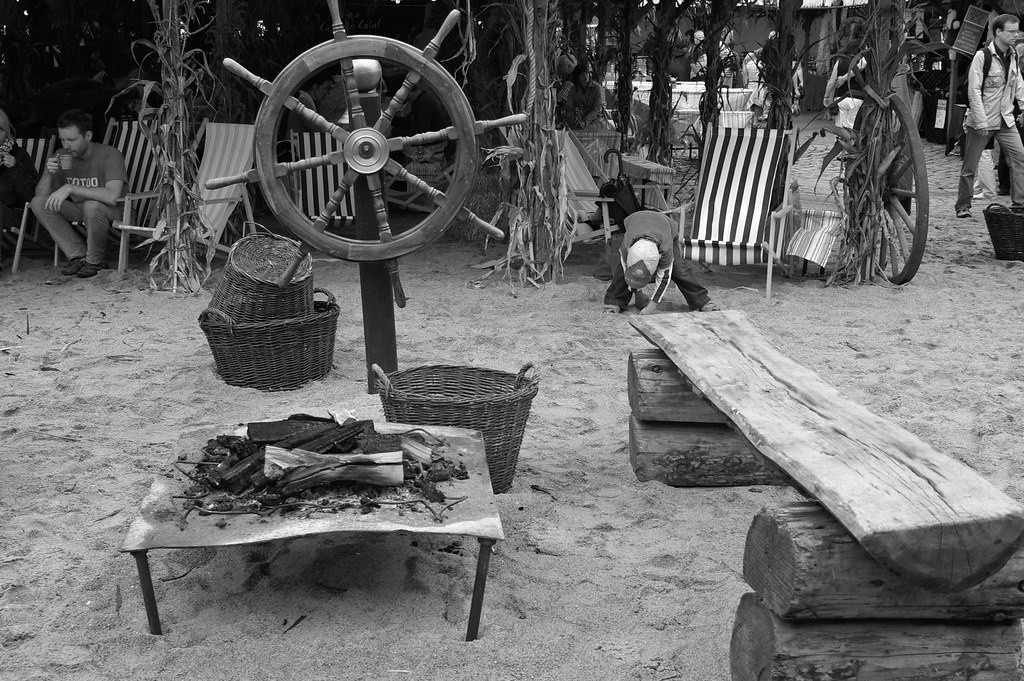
[[961, 47, 1019, 109]]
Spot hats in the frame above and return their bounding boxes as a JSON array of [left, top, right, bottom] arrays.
[[624, 240, 660, 289]]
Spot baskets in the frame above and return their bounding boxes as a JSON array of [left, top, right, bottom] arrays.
[[200, 221, 340, 393], [372, 363, 542, 493], [983, 203, 1024, 261]]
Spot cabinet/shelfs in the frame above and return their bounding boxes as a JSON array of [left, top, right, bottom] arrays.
[[620, 153, 675, 214], [571, 126, 622, 180]]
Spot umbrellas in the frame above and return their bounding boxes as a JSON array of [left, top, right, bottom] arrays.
[[594, 147, 642, 234]]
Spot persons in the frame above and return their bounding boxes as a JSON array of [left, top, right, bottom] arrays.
[[834, 28, 878, 88], [603, 209, 717, 318], [0, 108, 40, 272], [955, 13, 1024, 218], [565, 63, 604, 127], [668, 27, 803, 130], [30, 108, 130, 277], [823, 38, 867, 161]]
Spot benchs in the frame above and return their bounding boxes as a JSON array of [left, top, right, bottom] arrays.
[[627, 308, 1024, 681]]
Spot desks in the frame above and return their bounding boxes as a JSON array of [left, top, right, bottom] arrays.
[[119, 421, 506, 640]]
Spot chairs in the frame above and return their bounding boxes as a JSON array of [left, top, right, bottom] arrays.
[[290, 128, 357, 225], [556, 120, 799, 297], [0, 116, 258, 275]]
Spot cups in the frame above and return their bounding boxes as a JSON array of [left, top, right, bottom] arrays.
[[57, 154, 73, 169], [0, 148, 9, 165]]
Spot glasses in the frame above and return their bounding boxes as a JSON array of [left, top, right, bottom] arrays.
[[1002, 30, 1020, 35]]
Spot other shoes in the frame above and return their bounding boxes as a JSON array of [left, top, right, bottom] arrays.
[[957, 209, 971, 218], [602, 303, 620, 314], [700, 301, 720, 312], [77, 261, 108, 278], [973, 193, 996, 201], [61, 256, 87, 274]]
